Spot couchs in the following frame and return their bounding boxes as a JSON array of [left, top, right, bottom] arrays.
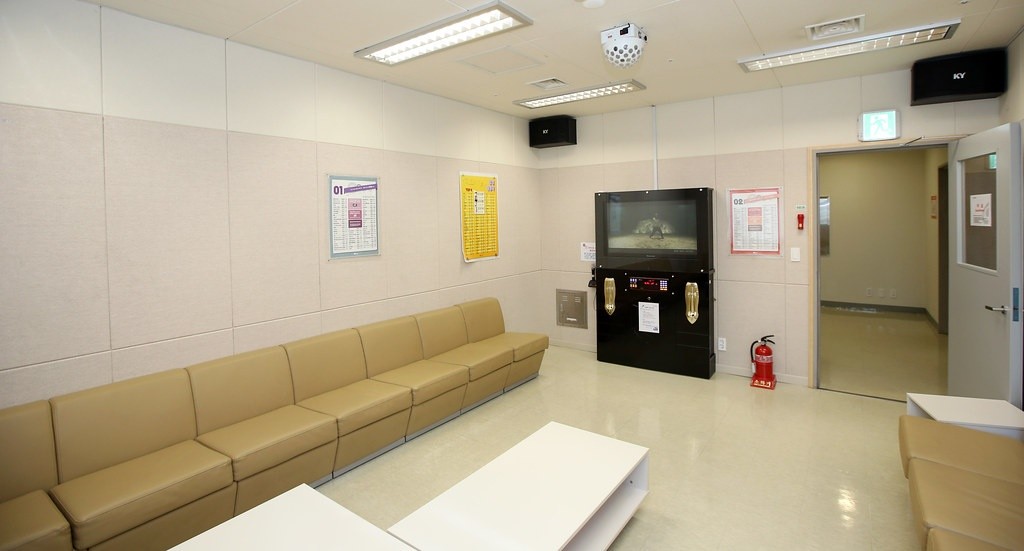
[[0, 295, 548, 551], [900, 413, 1024, 551]]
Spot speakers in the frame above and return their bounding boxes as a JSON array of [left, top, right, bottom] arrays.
[[529, 115, 577, 149], [910, 47, 1009, 106]]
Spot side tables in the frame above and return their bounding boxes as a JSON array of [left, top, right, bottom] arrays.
[[906, 392, 1024, 441]]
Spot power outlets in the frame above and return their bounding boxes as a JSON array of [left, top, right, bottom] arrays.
[[718, 338, 727, 351]]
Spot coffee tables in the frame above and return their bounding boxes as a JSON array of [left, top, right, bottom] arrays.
[[166, 422, 649, 551]]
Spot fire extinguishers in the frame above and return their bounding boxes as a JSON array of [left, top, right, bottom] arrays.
[[749, 334, 777, 390]]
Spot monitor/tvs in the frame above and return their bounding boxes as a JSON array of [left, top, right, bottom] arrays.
[[595, 187, 714, 274]]
